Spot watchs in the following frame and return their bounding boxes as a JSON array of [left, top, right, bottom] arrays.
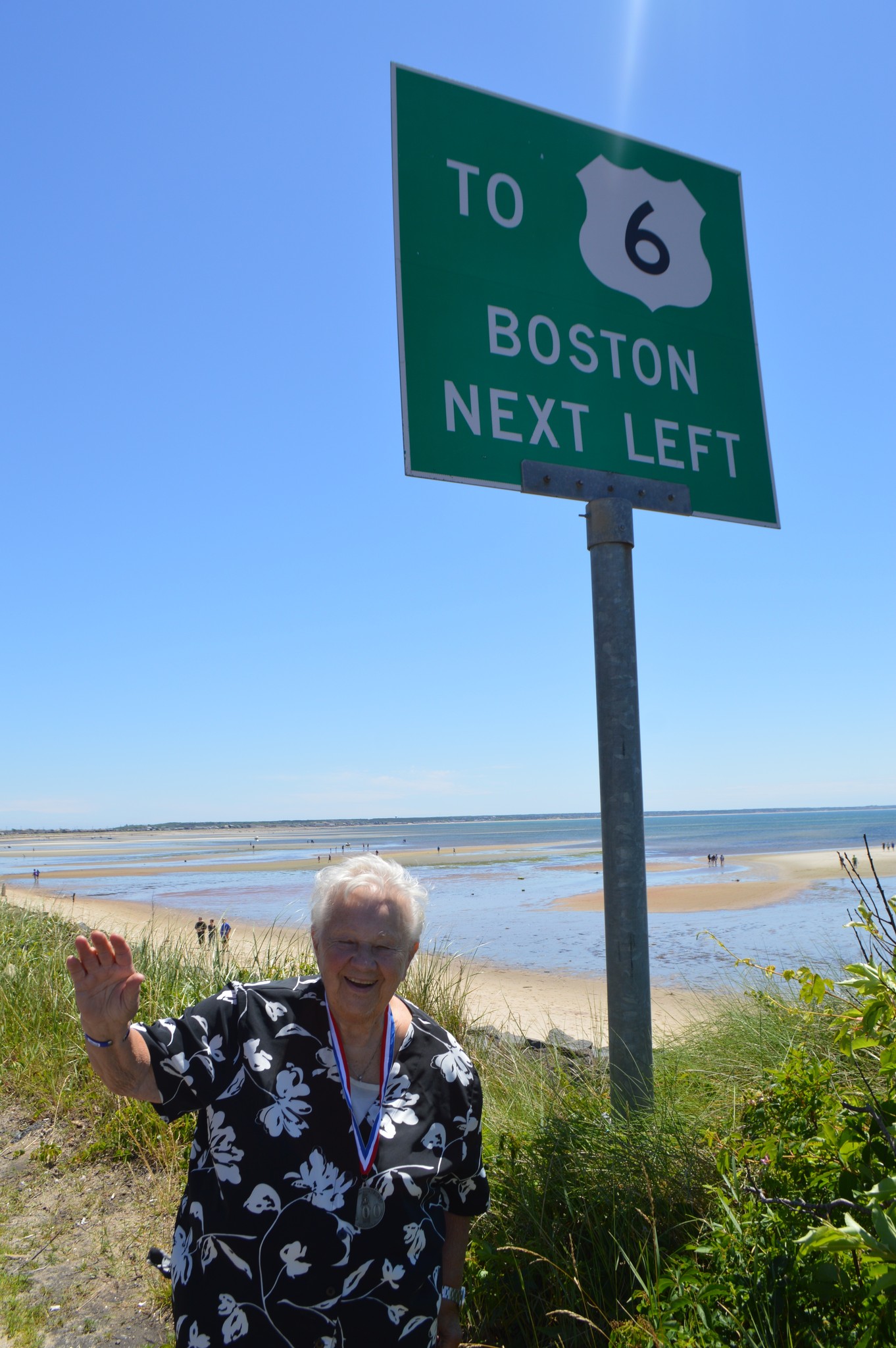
[[440, 1283, 466, 1311]]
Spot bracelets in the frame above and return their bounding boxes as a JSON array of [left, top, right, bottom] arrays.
[[84, 1018, 132, 1048]]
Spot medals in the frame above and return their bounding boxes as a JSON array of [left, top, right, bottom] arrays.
[[352, 1186, 387, 1230]]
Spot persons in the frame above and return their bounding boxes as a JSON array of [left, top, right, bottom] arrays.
[[68, 856, 492, 1347], [36, 870, 40, 880], [882, 841, 896, 851], [219, 918, 232, 953], [208, 919, 216, 948], [250, 836, 457, 863], [32, 869, 37, 880], [195, 916, 207, 946], [707, 853, 726, 867]]
